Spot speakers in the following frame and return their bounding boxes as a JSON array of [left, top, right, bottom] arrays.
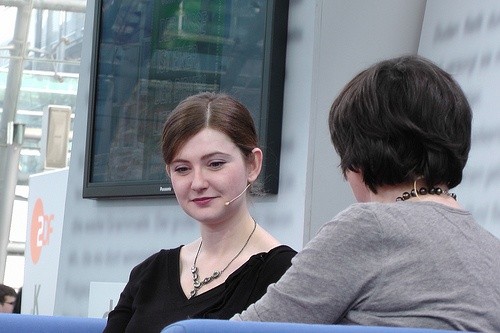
[[40, 104, 72, 170]]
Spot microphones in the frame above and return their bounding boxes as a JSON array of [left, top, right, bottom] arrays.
[[225, 182, 252, 206]]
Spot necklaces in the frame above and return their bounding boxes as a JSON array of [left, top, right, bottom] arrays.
[[190, 217, 256, 298], [396, 187, 457, 202]]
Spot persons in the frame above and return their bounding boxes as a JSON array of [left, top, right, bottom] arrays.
[[0, 284, 17, 313], [103, 91, 298, 333], [229, 56, 500, 333]]
